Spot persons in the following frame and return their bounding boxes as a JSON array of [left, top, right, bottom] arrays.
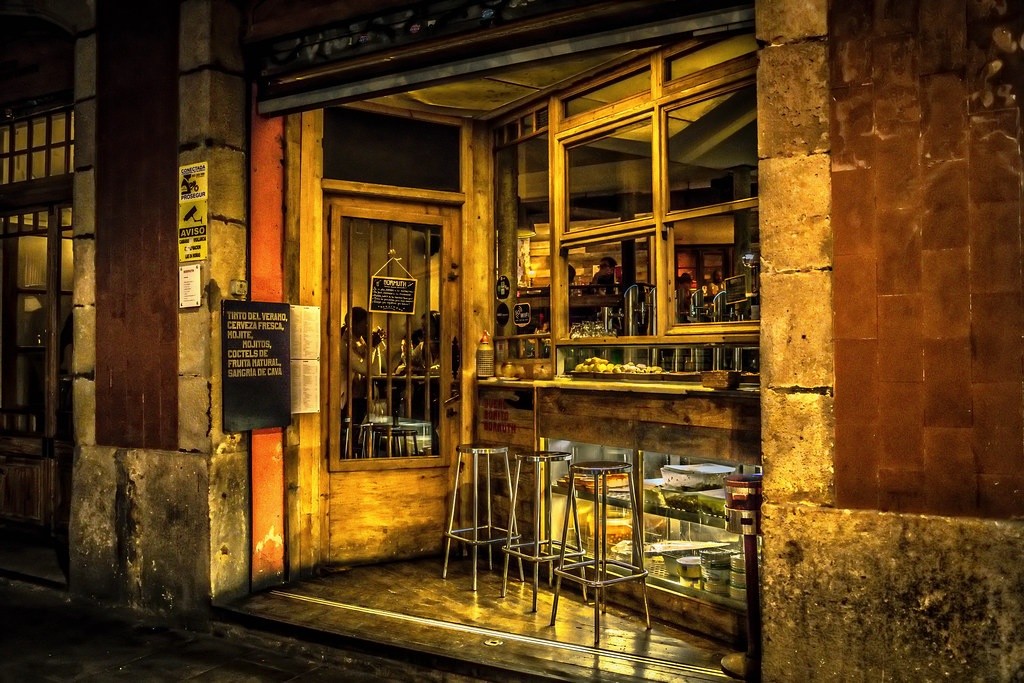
[[713, 271, 726, 290], [402, 309, 441, 455], [568, 264, 576, 285], [340, 307, 386, 458], [679, 271, 693, 303], [19, 307, 73, 441], [592, 257, 617, 295], [395, 334, 419, 375]]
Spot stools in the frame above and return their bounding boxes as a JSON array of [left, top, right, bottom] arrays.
[[342, 417, 420, 458], [501, 450, 590, 614], [549, 461, 653, 644], [439, 442, 525, 592]]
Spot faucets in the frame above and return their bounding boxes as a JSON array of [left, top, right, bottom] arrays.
[[713, 291, 726, 312], [689, 289, 701, 316]]
